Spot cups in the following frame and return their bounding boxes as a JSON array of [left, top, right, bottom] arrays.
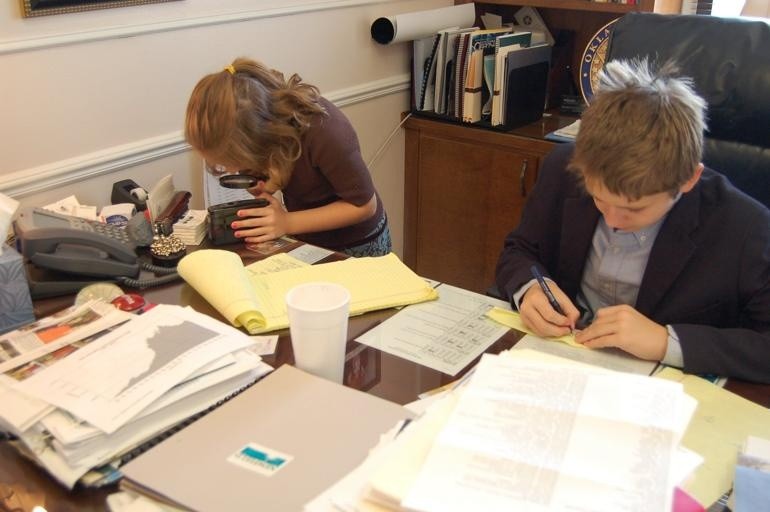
[[285, 285, 350, 384]]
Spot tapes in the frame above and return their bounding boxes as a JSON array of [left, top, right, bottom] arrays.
[[130, 187, 147, 201]]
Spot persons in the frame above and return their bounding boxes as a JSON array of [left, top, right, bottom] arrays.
[[184, 57, 392, 258], [494, 60, 770, 384]]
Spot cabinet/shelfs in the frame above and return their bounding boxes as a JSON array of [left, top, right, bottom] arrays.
[[405, 126, 541, 304]]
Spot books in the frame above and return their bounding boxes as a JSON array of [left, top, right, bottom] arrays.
[[412, 27, 550, 128]]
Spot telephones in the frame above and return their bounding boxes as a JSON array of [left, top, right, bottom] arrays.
[[11, 203, 140, 297]]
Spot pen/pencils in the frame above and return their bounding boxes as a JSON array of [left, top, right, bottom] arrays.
[[530, 265, 576, 335]]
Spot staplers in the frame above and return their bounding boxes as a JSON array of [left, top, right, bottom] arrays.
[[155, 190, 192, 235]]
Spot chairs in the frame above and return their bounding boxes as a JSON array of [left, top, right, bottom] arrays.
[[606, 10, 770, 211]]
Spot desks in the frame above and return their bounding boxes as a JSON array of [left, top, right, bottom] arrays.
[[0, 233, 769, 511]]
